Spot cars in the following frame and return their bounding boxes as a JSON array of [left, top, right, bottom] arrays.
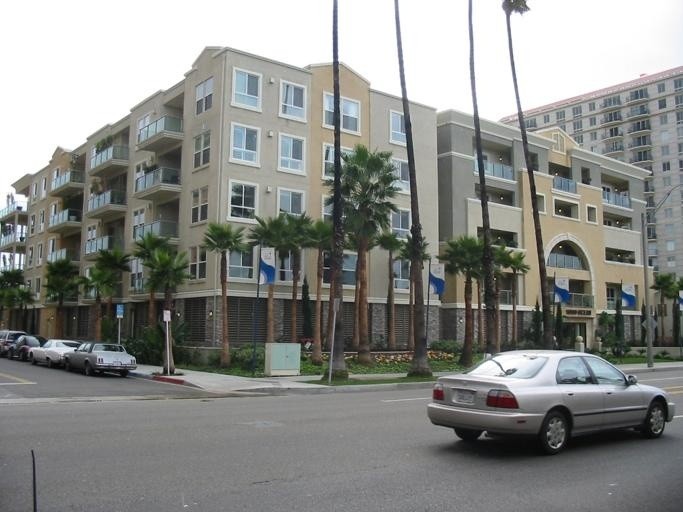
[[428, 349, 675, 455], [0, 331, 137, 377]]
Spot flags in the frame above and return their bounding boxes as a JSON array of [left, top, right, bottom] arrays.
[[554, 276, 569, 302], [429, 263, 445, 296], [259, 247, 276, 286], [678, 289, 682, 312], [621, 282, 636, 307]]
